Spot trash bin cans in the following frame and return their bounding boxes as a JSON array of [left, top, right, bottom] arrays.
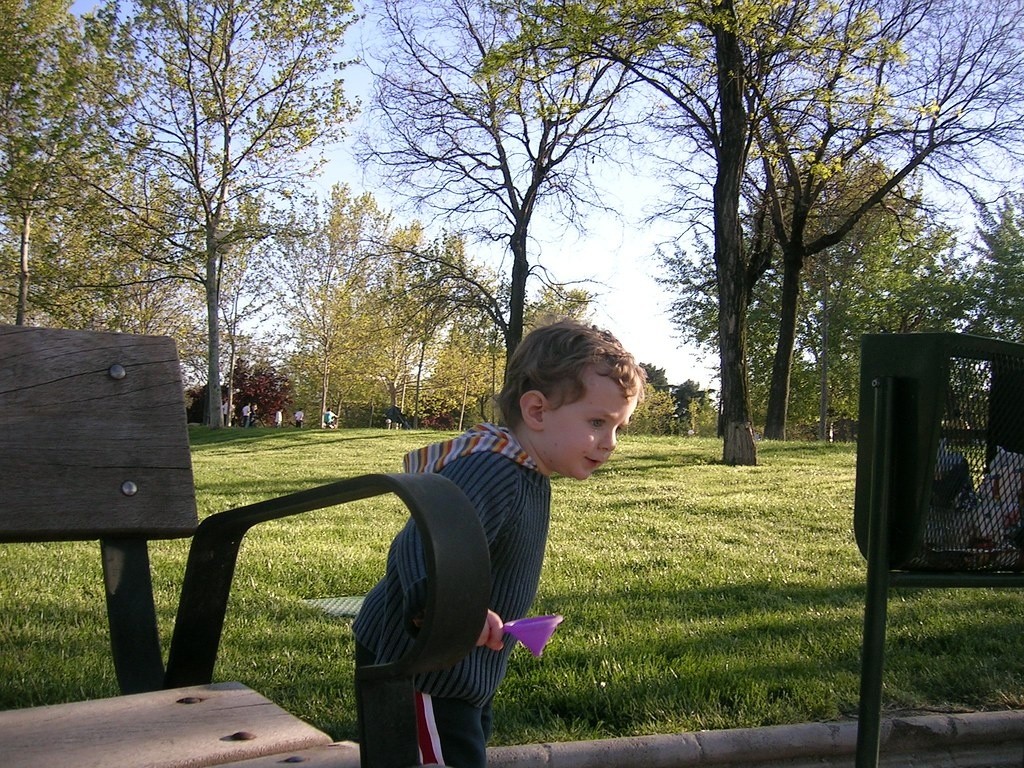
[[853, 332, 1024, 572], [392, 423, 398, 430]]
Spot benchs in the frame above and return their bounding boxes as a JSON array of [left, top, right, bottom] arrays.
[[1, 328, 492, 768]]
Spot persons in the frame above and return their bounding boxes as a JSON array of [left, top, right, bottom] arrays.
[[385, 417, 392, 424], [223, 399, 228, 415], [951, 408, 970, 429], [232, 412, 237, 427], [242, 403, 257, 427], [293, 408, 304, 427], [325, 408, 339, 429], [350, 318, 647, 768], [274, 409, 284, 427]]
